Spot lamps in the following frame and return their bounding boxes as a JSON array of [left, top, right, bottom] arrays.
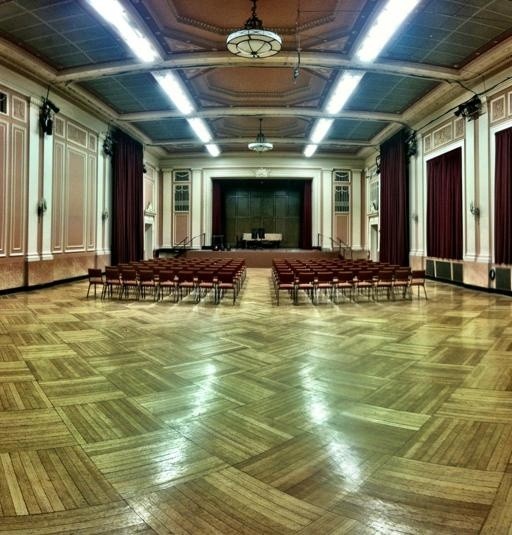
[[248, 117, 274, 153], [226, 0, 283, 58]]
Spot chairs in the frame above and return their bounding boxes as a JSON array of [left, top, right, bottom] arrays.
[[87, 256, 247, 307], [269, 257, 427, 306]]
